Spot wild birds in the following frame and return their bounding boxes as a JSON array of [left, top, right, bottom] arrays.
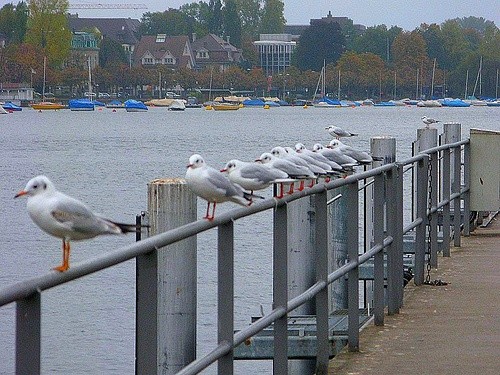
[[421, 116, 441, 129], [325, 125, 359, 140], [13, 175, 150, 271], [184, 137, 374, 222]]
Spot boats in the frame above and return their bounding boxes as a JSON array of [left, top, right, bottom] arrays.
[[2, 102, 22, 111], [69, 97, 500, 112], [31, 104, 64, 109]]
